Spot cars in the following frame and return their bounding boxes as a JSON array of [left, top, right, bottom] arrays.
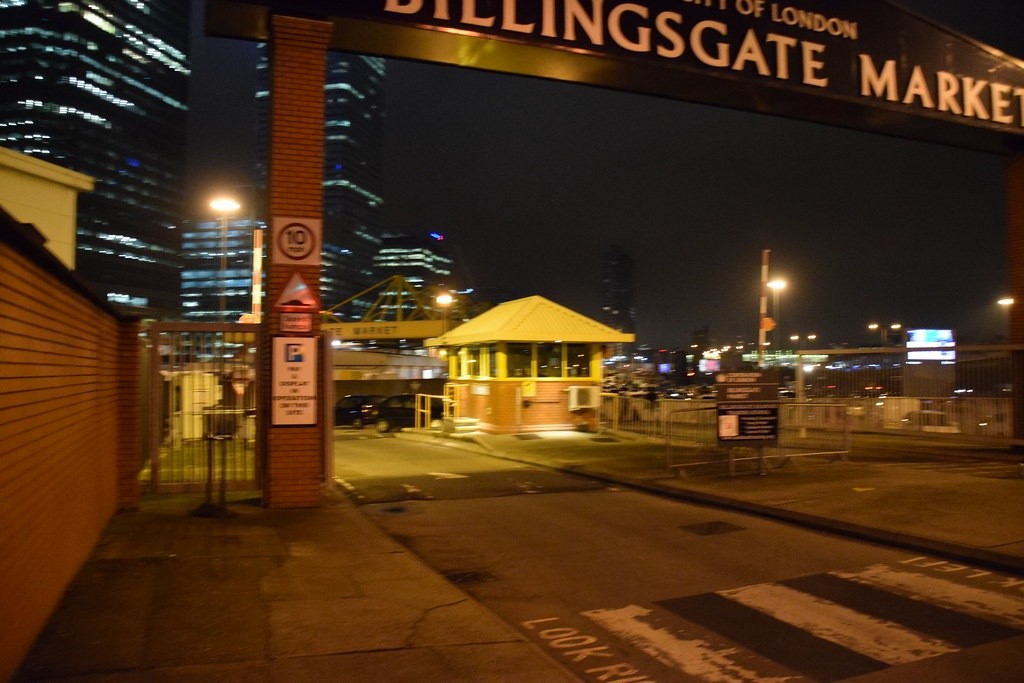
[[899, 410, 960, 433], [780, 389, 978, 416], [334, 394, 386, 429], [602, 377, 716, 399], [825, 362, 847, 369], [362, 394, 454, 433]]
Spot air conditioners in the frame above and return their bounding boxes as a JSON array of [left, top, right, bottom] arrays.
[[568, 385, 601, 408]]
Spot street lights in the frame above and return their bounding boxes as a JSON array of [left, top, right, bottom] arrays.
[[767, 280, 783, 351], [436, 296, 450, 333], [211, 198, 239, 320], [868, 323, 901, 347], [791, 335, 817, 349]]
